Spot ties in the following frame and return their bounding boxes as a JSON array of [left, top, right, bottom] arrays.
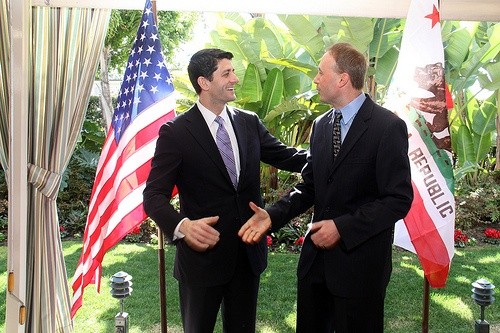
[[214, 116, 238, 191], [333, 113, 343, 160]]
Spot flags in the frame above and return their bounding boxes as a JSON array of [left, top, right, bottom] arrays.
[[69, 0, 178, 318], [383, 0, 456, 289]]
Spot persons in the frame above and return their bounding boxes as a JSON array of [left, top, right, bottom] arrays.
[[238, 43, 414, 333], [143, 49, 308, 333]]
